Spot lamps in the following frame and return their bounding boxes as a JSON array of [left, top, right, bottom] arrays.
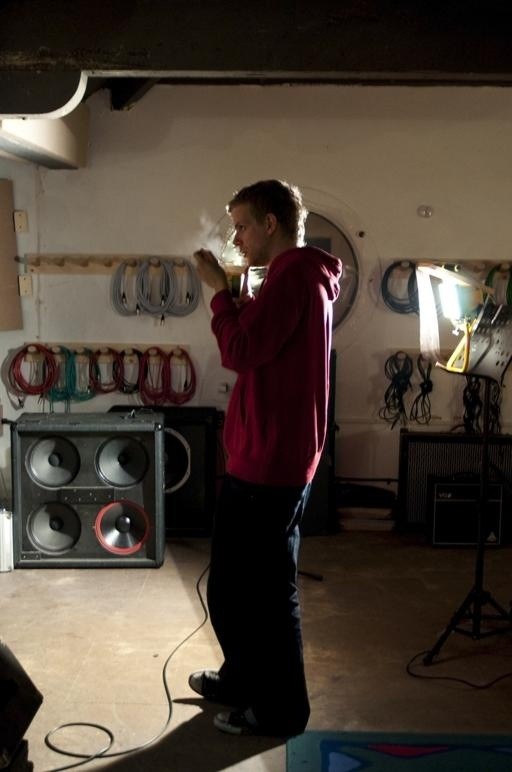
[[437, 281, 483, 321]]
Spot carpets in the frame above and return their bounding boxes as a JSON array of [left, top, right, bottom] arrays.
[[286, 730, 512, 772]]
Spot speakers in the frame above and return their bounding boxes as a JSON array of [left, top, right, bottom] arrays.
[[12, 411, 166, 569], [111, 405, 215, 540]]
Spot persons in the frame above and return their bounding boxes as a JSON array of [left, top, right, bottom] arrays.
[[188, 180, 344, 736]]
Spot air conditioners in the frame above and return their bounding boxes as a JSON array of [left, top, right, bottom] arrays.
[[0, 103, 90, 170]]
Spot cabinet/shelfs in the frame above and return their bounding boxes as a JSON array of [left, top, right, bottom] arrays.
[[426, 473, 505, 549]]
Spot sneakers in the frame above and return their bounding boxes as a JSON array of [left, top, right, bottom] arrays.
[[214, 706, 259, 733], [189, 670, 219, 700]]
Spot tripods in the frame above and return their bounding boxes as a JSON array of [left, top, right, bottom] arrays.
[[422, 378, 512, 666]]
